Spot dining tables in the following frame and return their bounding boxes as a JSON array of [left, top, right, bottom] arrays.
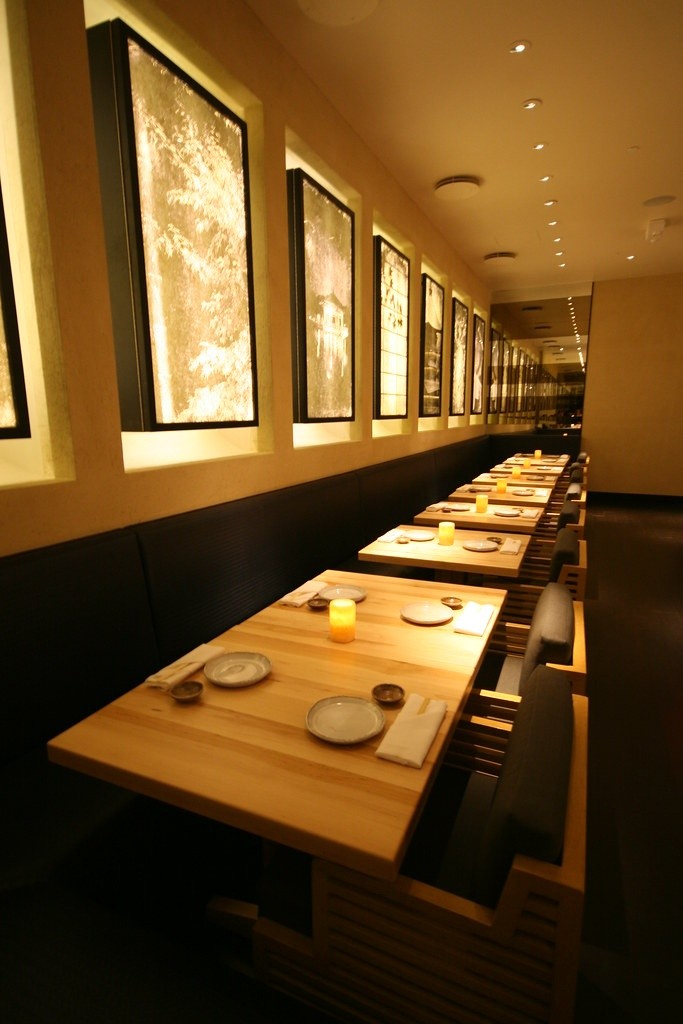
[[45, 564, 508, 975], [357, 453, 568, 589]]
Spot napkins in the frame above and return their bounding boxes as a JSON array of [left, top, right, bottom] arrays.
[[500, 538, 520, 554], [545, 476, 555, 481], [535, 489, 547, 497], [479, 474, 490, 479], [377, 529, 404, 542], [278, 579, 327, 608], [372, 694, 447, 769], [142, 643, 224, 691], [426, 503, 448, 512], [451, 599, 495, 637], [521, 509, 538, 519], [456, 485, 470, 492]]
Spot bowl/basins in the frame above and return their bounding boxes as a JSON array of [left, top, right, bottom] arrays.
[[469, 490, 476, 492], [371, 684, 405, 700], [440, 596, 463, 606], [512, 508, 523, 512], [442, 508, 450, 512], [170, 681, 203, 701], [307, 598, 327, 607], [398, 538, 408, 543], [487, 537, 501, 542]]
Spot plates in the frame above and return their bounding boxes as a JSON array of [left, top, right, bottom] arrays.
[[491, 476, 508, 477], [494, 510, 518, 516], [491, 454, 559, 470], [306, 696, 385, 744], [203, 652, 271, 687], [527, 479, 543, 480], [405, 530, 434, 540], [447, 504, 469, 510], [402, 600, 453, 624], [319, 585, 366, 601], [512, 490, 532, 495], [463, 539, 498, 551], [469, 485, 491, 491]]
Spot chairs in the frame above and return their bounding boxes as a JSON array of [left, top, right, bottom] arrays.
[[465, 451, 593, 721], [202, 692, 591, 1024]]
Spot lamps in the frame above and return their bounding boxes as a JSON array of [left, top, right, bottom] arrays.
[[297, 0, 378, 29], [484, 251, 515, 268], [434, 175, 479, 202], [521, 307, 566, 361]]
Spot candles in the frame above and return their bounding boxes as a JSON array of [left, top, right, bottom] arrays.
[[328, 598, 358, 643], [523, 459, 531, 469], [534, 449, 542, 459], [475, 494, 488, 513], [438, 522, 455, 546], [496, 479, 507, 494], [511, 468, 521, 479]]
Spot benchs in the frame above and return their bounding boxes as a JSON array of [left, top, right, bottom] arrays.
[[131, 430, 502, 657], [0, 524, 171, 896]]
